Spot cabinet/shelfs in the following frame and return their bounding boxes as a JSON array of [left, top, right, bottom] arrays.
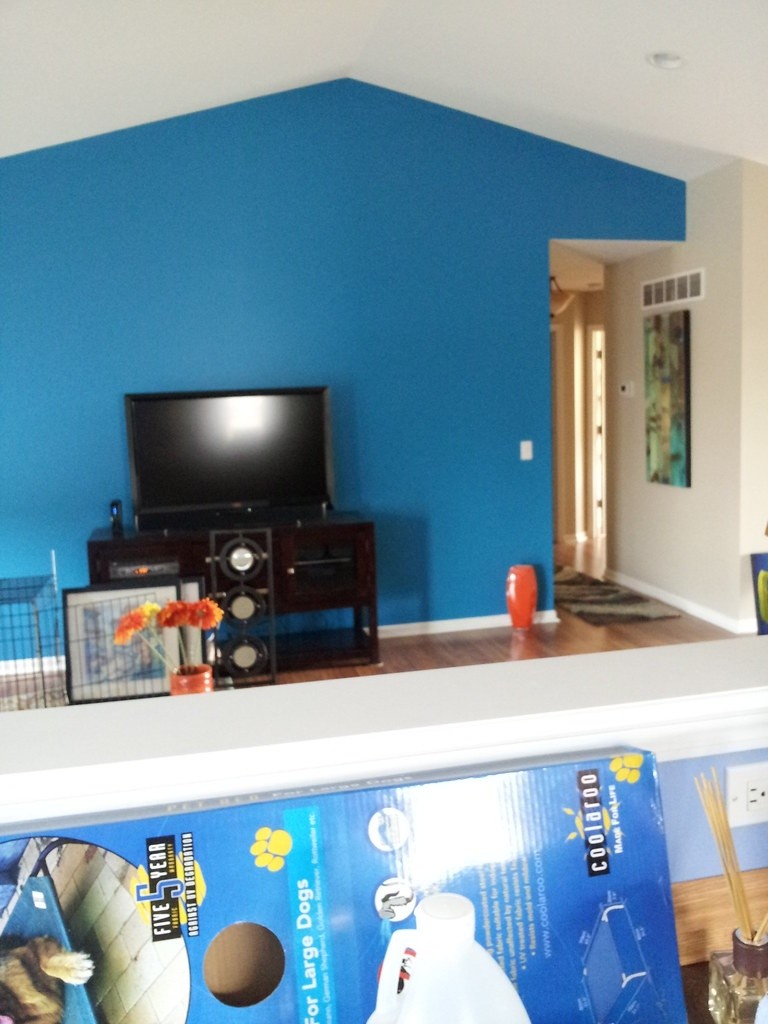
[[87, 508, 380, 673]]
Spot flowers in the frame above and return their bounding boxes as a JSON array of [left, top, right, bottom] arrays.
[[114, 598, 225, 674]]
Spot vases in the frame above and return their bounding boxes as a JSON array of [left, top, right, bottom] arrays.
[[170, 662, 212, 697]]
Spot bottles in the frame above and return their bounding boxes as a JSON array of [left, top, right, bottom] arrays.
[[366, 893, 529, 1024], [708, 928, 768, 1024]]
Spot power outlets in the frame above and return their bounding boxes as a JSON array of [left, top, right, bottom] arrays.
[[725, 761, 768, 829]]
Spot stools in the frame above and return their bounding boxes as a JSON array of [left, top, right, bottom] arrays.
[[1, 574, 64, 710]]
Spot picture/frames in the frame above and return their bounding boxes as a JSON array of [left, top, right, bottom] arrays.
[[642, 308, 692, 487], [180, 575, 208, 666], [61, 576, 185, 705]]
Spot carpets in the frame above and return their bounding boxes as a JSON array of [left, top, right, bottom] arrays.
[[554, 564, 683, 630]]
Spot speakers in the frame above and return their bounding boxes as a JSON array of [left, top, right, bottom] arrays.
[[208, 525, 278, 688]]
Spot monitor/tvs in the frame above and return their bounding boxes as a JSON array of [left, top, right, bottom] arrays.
[[124, 386, 333, 533]]
